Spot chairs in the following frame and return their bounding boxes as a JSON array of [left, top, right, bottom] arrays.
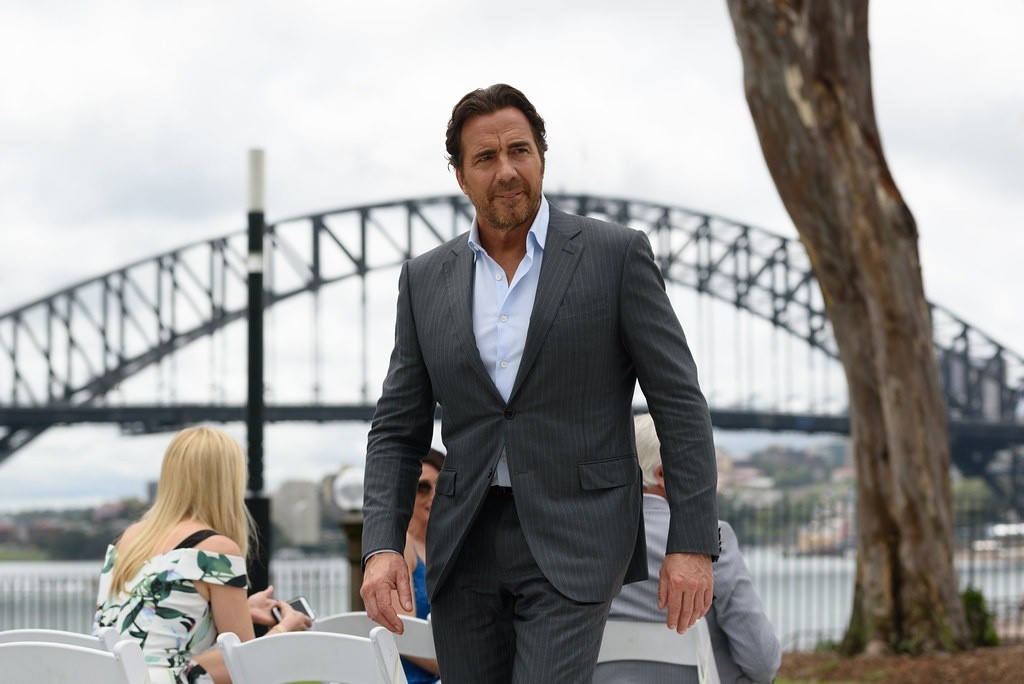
[[0, 610, 720, 684]]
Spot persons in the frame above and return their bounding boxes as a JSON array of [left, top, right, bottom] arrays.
[[395, 412, 780, 684], [92, 425, 312, 684], [360, 85, 723, 684]]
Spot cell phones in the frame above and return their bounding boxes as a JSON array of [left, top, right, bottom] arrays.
[[271, 597, 316, 625]]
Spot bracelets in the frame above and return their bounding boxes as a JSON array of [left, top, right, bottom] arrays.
[[273, 626, 287, 633]]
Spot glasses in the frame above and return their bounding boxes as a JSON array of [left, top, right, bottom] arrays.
[[418, 481, 435, 493]]
